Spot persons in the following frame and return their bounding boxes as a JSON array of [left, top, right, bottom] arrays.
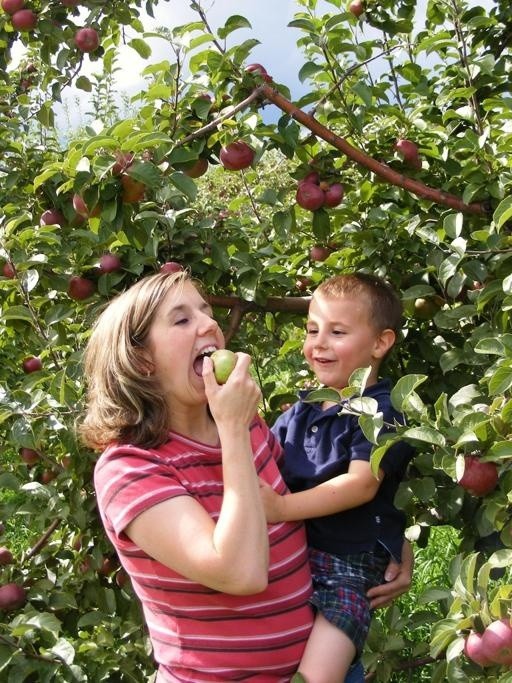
[[256, 274, 416, 683], [78, 272, 414, 683]]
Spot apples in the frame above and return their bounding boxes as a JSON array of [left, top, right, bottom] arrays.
[[458, 457, 498, 498], [0, 521, 131, 612], [68, 275, 96, 300], [21, 446, 73, 484], [0, 0, 99, 54], [308, 245, 328, 262], [209, 350, 238, 385], [389, 263, 480, 319], [160, 262, 183, 275], [294, 279, 309, 291], [100, 255, 121, 273], [23, 357, 42, 374], [462, 618, 512, 668], [349, 0, 362, 17], [40, 63, 273, 227], [296, 139, 422, 211], [3, 263, 15, 278]]
